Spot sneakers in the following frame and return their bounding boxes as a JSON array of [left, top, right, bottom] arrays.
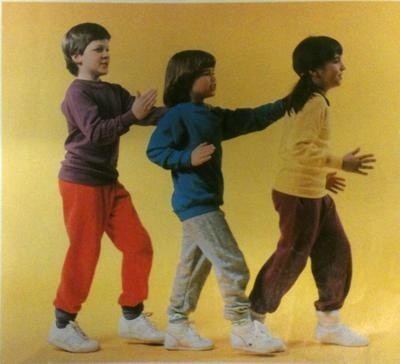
[[317, 324, 369, 346], [231, 321, 286, 354], [166, 321, 213, 351], [48, 320, 99, 353], [120, 311, 165, 344]]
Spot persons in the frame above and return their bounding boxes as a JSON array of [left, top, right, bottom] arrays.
[[248, 35, 377, 347], [45, 23, 174, 354], [145, 50, 290, 356]]
